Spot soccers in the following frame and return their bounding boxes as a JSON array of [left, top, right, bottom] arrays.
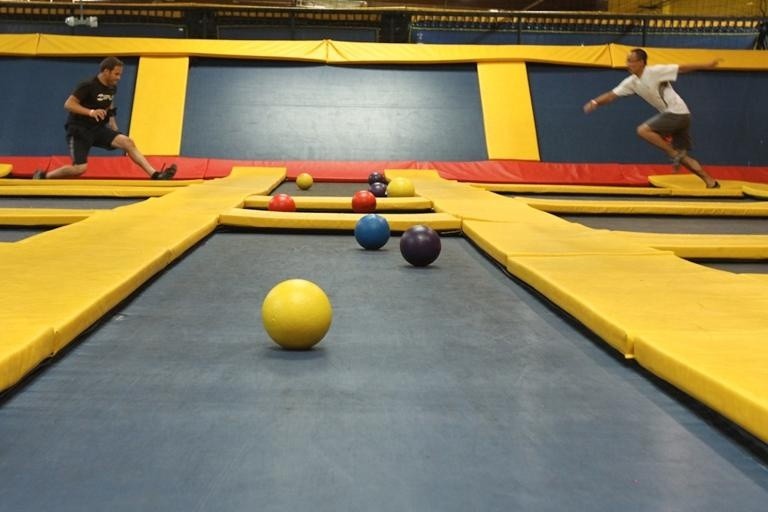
[[296, 172, 314, 190], [262, 280, 332, 349], [369, 172, 415, 197], [269, 194, 297, 213], [355, 214, 391, 250], [352, 190, 376, 213], [400, 226, 441, 266]]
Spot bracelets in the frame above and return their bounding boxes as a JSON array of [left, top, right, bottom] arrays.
[[592, 98, 599, 106], [89, 108, 94, 117]]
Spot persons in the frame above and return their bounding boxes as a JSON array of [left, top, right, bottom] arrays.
[[584, 48, 728, 190], [32, 55, 178, 180]]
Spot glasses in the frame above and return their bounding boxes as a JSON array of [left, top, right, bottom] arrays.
[[627, 58, 640, 64]]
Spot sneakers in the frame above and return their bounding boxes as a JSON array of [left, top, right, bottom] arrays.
[[33, 170, 42, 179], [706, 180, 720, 188], [671, 150, 687, 171], [157, 163, 177, 180]]
